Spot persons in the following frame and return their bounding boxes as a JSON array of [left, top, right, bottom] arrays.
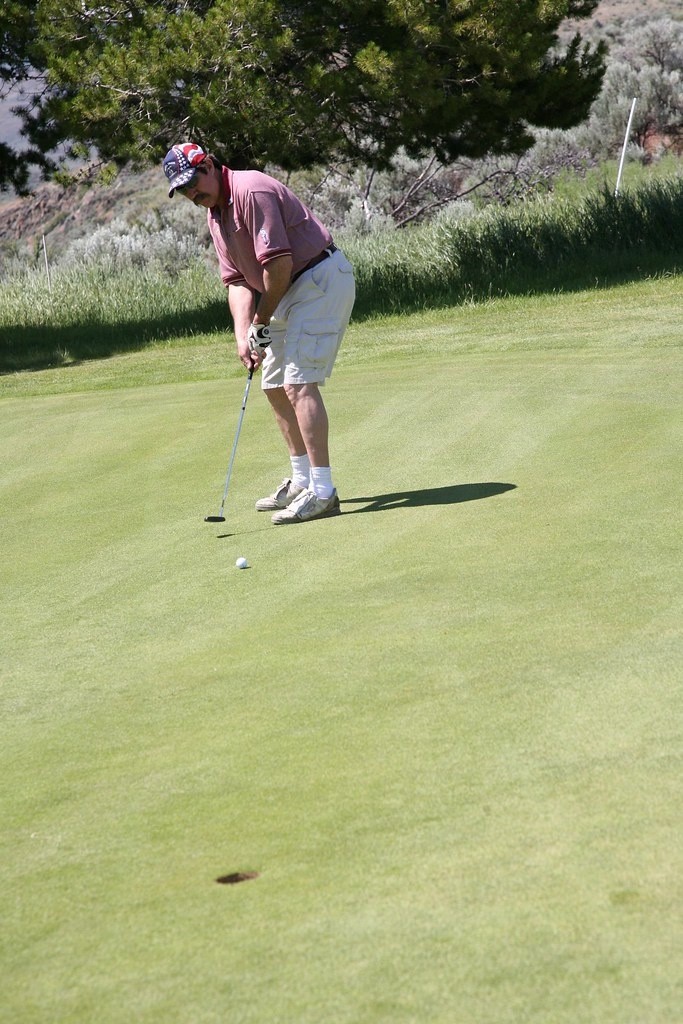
[[161, 142, 359, 526]]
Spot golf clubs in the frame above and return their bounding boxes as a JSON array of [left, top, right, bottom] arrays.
[[204, 356, 256, 524]]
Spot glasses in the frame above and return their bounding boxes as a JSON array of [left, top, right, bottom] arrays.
[[176, 170, 201, 195]]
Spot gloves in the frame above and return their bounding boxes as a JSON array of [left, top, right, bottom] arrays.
[[247, 322, 273, 355]]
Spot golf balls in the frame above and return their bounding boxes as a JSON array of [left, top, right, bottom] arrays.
[[235, 556, 248, 568]]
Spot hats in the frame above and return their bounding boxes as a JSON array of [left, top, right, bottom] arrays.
[[163, 143, 207, 198]]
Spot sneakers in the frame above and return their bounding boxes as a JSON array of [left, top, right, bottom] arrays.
[[255, 478, 307, 511], [271, 486, 340, 524]]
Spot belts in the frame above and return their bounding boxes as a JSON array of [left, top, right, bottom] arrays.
[[292, 244, 338, 286]]
